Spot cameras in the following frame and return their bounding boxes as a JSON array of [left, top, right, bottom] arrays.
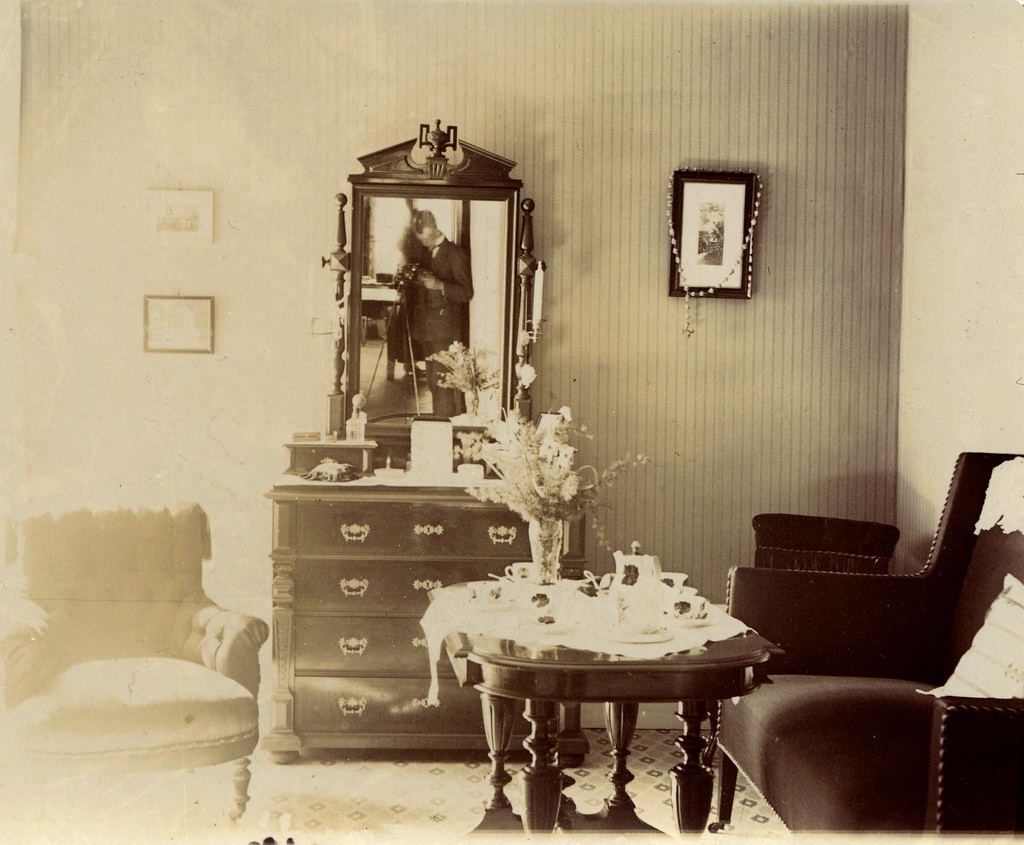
[[396, 262, 419, 284]]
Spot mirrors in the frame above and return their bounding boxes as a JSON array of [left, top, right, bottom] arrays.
[[321, 117, 548, 447]]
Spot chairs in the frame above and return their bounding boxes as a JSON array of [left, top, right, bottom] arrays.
[[0, 502, 271, 828]]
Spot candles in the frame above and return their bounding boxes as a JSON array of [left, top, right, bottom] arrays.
[[532, 261, 544, 320]]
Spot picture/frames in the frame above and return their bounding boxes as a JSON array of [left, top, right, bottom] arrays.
[[141, 293, 215, 355], [668, 170, 756, 300], [142, 188, 214, 247]]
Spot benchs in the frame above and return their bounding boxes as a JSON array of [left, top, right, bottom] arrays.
[[708, 451, 1024, 836]]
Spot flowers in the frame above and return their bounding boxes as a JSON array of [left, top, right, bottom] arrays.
[[453, 362, 650, 551], [424, 339, 500, 400]]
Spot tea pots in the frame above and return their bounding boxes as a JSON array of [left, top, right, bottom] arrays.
[[607, 540, 688, 643]]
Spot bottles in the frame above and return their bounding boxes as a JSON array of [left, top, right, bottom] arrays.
[[346, 408, 365, 442]]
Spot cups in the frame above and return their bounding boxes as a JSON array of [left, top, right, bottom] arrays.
[[468, 580, 501, 606], [504, 562, 539, 583], [530, 586, 574, 634], [669, 586, 707, 619]]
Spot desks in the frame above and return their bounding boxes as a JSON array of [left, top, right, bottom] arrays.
[[426, 580, 785, 839]]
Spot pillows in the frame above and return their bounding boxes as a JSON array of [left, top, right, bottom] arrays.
[[915, 573, 1024, 700]]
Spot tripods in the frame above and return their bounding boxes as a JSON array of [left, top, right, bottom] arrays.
[[368, 284, 419, 424]]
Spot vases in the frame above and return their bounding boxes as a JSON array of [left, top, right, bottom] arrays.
[[528, 519, 563, 587], [465, 392, 480, 417]]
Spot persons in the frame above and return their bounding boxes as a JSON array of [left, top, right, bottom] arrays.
[[408, 210, 473, 421]]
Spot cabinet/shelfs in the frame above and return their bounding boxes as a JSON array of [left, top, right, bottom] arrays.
[[260, 467, 590, 768]]
